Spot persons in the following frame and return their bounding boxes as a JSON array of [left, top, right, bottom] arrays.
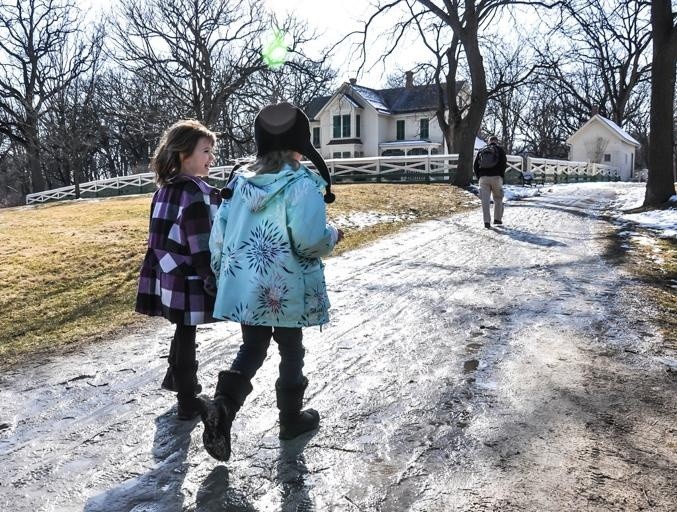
[[134, 119, 222, 420], [474, 136, 507, 228], [201, 101, 344, 461]]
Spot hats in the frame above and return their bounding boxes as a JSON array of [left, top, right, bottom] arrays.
[[254, 102, 310, 154]]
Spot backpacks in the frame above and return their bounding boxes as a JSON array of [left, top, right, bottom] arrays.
[[479, 146, 498, 168]]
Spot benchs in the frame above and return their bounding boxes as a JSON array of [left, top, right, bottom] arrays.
[[520, 171, 545, 188]]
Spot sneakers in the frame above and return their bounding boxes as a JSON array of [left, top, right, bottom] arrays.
[[485, 220, 502, 228]]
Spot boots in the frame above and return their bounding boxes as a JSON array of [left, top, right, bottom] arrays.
[[177, 387, 210, 421], [201, 370, 253, 461], [275, 377, 320, 440]]
